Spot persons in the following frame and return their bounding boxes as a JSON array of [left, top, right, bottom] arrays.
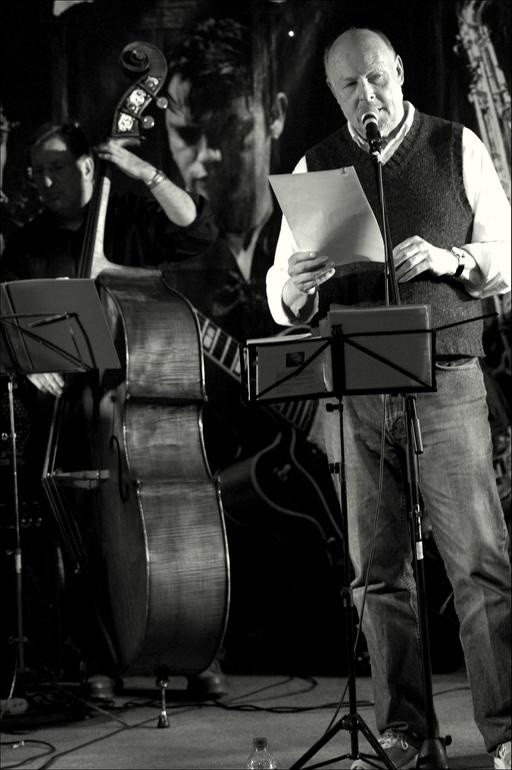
[[163, 18, 334, 489], [263, 27, 512, 770], [0, 122, 228, 701]]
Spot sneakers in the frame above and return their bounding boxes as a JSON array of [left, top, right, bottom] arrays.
[[494, 741, 511, 769], [351, 732, 429, 769]]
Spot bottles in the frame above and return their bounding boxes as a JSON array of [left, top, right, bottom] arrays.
[[244, 735, 282, 769]]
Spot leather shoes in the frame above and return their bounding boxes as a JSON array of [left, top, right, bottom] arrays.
[[188, 668, 227, 700], [81, 676, 114, 698]]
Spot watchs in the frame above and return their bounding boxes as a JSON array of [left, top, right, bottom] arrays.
[[452, 246, 467, 278]]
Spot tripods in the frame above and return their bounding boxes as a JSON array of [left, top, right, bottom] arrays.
[[287, 403, 403, 769], [1, 377, 129, 732]]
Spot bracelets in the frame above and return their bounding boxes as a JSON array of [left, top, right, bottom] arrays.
[[145, 167, 164, 189]]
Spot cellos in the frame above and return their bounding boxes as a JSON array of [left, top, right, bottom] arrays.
[[43, 40, 232, 677]]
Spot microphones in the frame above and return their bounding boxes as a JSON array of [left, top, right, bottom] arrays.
[[355, 104, 383, 155]]
